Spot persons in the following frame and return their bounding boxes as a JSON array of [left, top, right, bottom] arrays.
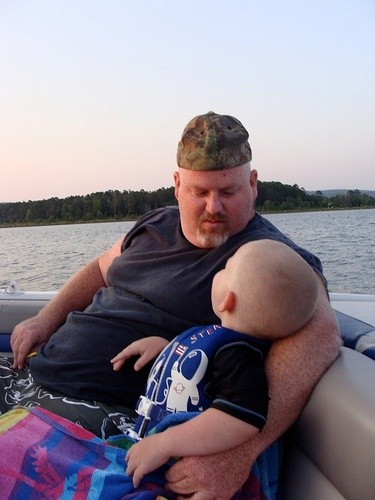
[[110, 238, 321, 500], [0, 111, 343, 500]]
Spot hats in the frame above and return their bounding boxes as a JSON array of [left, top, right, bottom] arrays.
[[176, 111, 253, 171]]
